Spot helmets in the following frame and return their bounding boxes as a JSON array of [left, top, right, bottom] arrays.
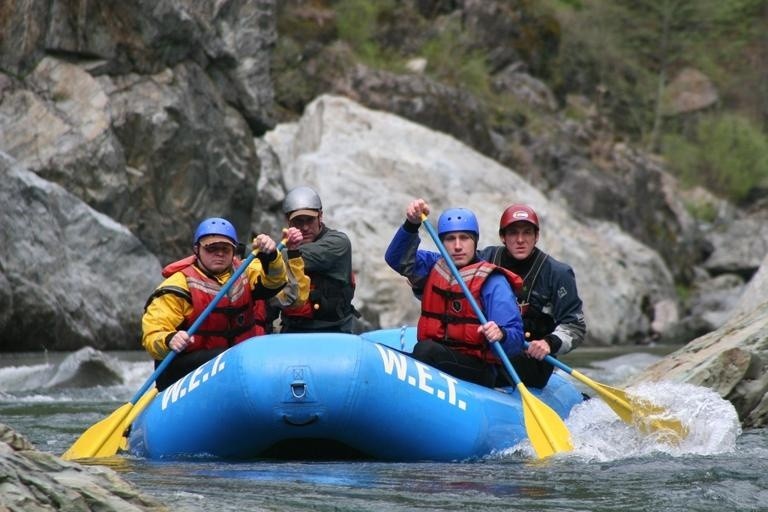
[[438, 207, 479, 236], [499, 204, 540, 231], [282, 186, 323, 213], [193, 218, 239, 244]]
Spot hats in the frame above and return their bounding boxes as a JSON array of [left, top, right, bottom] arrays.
[[287, 210, 320, 220], [198, 234, 237, 248]]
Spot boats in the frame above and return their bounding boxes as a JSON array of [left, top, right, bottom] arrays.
[[188, 459, 557, 497], [127, 325, 583, 462]]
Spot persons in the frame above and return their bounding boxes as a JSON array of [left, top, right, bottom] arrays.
[[143, 217, 288, 393], [475, 203, 586, 389], [384, 198, 526, 389], [233, 226, 310, 334], [276, 187, 372, 335]]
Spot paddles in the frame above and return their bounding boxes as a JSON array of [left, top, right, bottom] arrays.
[[420, 213, 575, 460], [60, 246, 261, 462], [523, 341, 688, 440], [115, 237, 288, 452]]
